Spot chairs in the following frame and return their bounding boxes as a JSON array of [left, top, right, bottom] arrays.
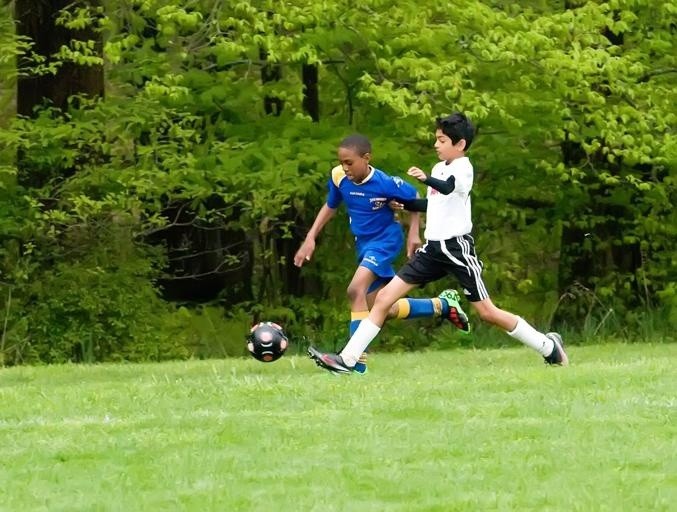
[[437, 289, 471, 336], [307, 347, 369, 377], [543, 332, 569, 367]]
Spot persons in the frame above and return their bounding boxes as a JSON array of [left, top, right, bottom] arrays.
[[304, 111, 570, 375], [291, 132, 471, 376]]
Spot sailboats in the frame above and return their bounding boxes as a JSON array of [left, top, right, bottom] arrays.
[[246, 322, 289, 362]]
